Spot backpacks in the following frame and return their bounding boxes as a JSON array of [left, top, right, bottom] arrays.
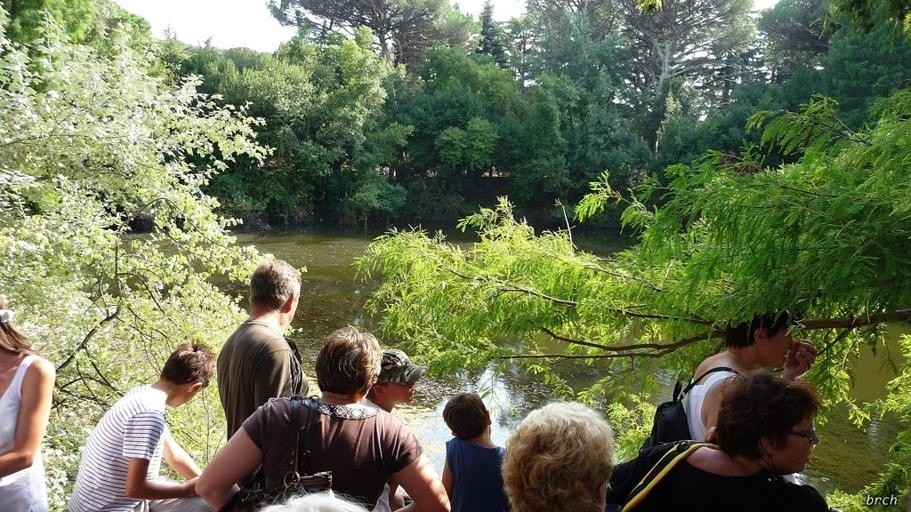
[[638, 366, 748, 457]]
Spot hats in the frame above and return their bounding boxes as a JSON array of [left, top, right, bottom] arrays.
[[373, 348, 429, 386]]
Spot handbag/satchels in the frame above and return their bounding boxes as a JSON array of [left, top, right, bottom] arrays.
[[217, 468, 333, 512]]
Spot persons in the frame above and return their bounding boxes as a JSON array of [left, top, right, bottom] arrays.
[[64, 337, 241, 512], [440, 391, 512, 510], [603, 371, 837, 510], [0, 295, 56, 512], [680, 306, 818, 482], [358, 345, 427, 510], [500, 400, 614, 511], [215, 261, 306, 443], [192, 323, 454, 512]]
